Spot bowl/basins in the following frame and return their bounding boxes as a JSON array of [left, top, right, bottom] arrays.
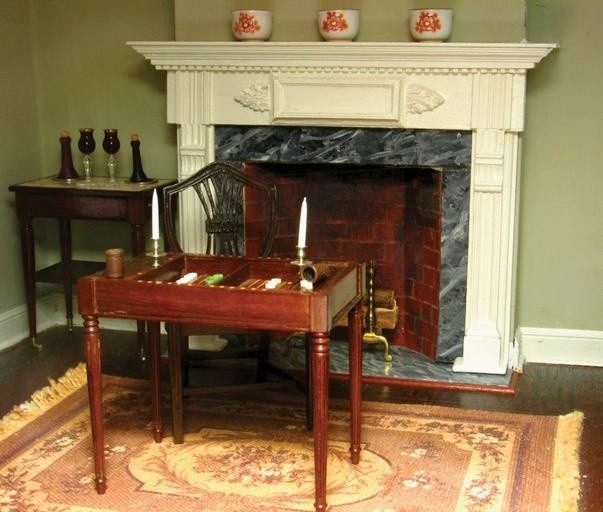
[[317, 8, 360, 42], [407, 7, 454, 42], [229, 9, 272, 42]]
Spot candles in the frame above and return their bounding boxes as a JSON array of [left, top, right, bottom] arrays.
[[290, 195, 313, 248], [145, 188, 167, 237]]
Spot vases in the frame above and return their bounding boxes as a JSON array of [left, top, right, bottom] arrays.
[[231, 7, 455, 43]]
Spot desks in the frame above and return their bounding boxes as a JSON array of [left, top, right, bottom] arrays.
[[75, 248, 365, 512], [7, 174, 178, 364]]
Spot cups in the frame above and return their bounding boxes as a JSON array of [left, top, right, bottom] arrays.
[[300, 264, 329, 284], [104, 248, 124, 279]]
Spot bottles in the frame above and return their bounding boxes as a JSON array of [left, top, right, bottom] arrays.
[[128, 135, 148, 183], [57, 132, 79, 179]]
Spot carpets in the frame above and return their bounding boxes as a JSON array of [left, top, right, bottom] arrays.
[[0, 360, 584, 511]]
